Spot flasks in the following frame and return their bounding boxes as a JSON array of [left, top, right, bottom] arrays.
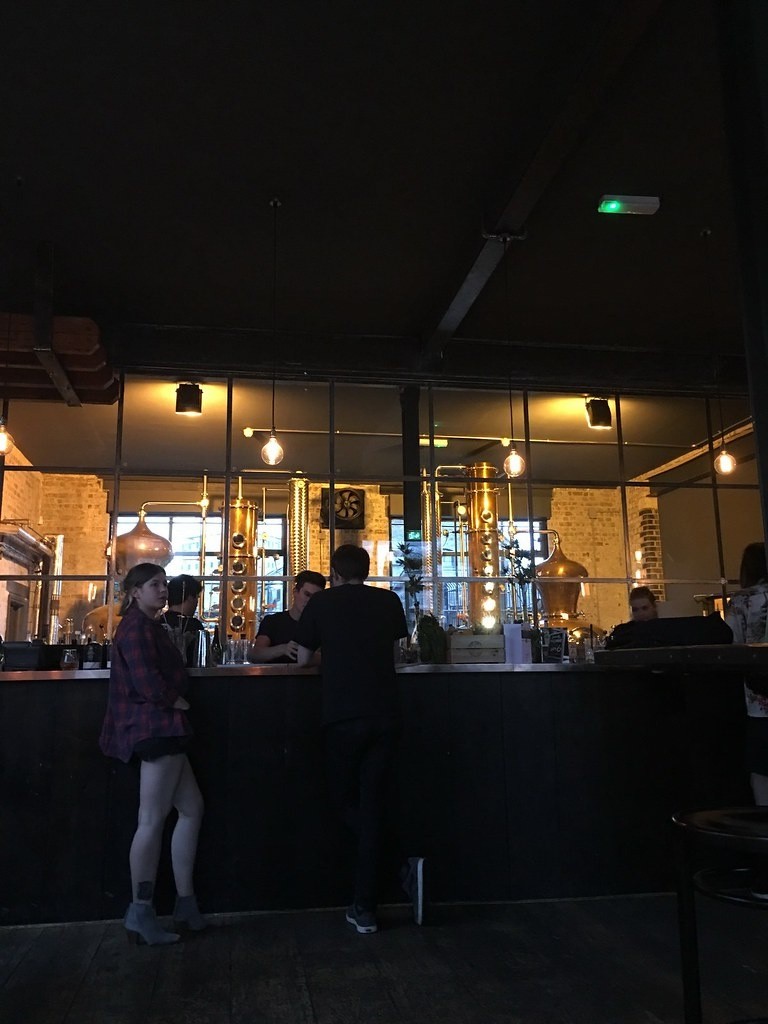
[[191, 630, 211, 668]]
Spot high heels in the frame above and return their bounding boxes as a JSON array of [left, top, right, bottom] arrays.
[[176, 895, 216, 932], [124, 901, 183, 949]]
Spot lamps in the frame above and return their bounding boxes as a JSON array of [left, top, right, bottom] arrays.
[[502, 388, 525, 475], [262, 375, 283, 465], [175, 380, 204, 415], [715, 396, 736, 475], [582, 395, 614, 429]]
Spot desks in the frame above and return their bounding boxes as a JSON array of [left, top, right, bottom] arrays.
[[594, 640, 768, 677]]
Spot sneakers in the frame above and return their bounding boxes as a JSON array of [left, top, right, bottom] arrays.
[[345, 899, 380, 934], [397, 855, 428, 926]]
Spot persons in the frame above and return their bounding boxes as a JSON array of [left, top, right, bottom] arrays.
[[254, 570, 326, 664], [98, 562, 204, 947], [629, 587, 658, 622], [292, 544, 431, 933], [725, 541, 768, 806], [159, 575, 204, 666]]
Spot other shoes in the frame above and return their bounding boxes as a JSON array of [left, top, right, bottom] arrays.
[[750, 884, 768, 900]]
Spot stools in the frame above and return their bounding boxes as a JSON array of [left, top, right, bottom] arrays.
[[670, 803, 768, 1024]]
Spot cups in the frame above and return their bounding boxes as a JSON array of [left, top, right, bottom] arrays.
[[60, 649, 79, 671], [584, 637, 597, 662]]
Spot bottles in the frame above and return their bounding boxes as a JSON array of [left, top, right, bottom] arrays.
[[83, 633, 103, 669], [211, 625, 221, 664], [223, 634, 257, 665]]
